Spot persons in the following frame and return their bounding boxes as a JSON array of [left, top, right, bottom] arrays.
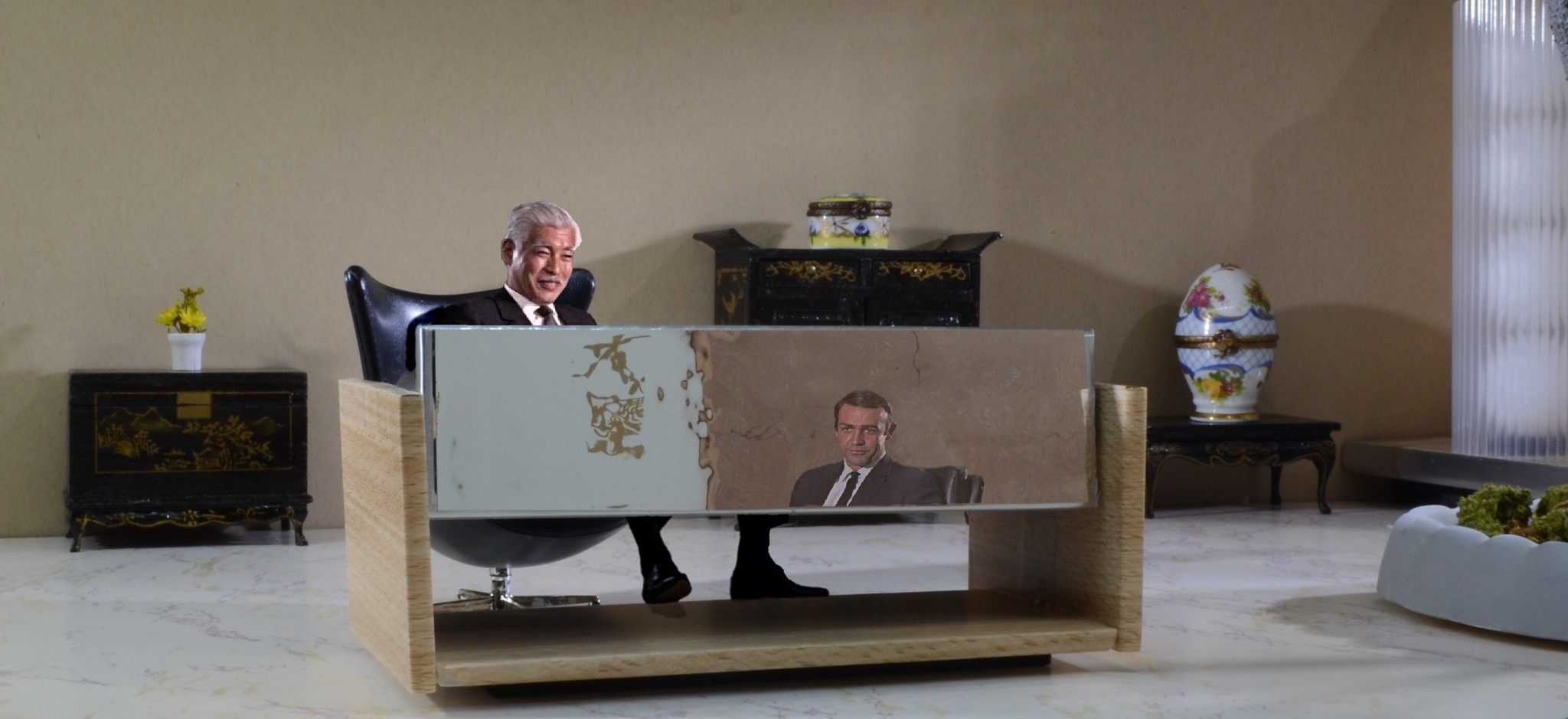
[[405, 200, 831, 602], [789, 391, 942, 508]]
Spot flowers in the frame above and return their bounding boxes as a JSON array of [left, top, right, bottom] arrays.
[[155, 287, 207, 334]]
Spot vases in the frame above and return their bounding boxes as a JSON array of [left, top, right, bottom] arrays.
[[168, 333, 205, 373]]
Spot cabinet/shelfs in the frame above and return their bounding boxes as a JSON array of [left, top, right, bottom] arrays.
[[693, 226, 1003, 519], [63, 369, 314, 553]]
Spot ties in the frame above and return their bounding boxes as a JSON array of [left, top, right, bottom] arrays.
[[537, 306, 559, 326], [835, 471, 861, 506]]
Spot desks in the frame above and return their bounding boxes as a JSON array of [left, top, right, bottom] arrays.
[[1146, 413, 1342, 520]]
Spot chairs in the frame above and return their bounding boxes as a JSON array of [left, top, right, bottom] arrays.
[[344, 265, 628, 612]]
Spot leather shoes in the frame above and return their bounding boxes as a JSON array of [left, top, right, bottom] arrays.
[[641, 565, 692, 605], [730, 560, 829, 596]]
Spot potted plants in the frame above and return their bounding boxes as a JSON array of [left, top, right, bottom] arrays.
[[1377, 481, 1568, 642]]
